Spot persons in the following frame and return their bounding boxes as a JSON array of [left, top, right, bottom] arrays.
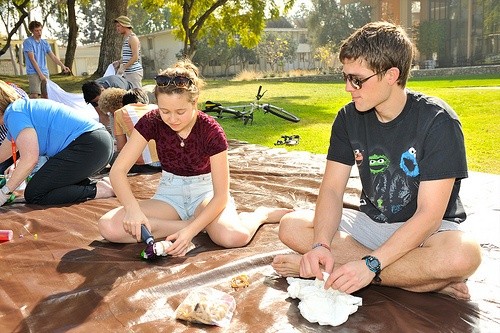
[[109, 88, 162, 175], [98, 88, 127, 139], [272, 20, 482, 300], [82, 75, 138, 130], [112, 16, 143, 90], [23, 21, 71, 99], [0, 80, 116, 206], [98, 58, 294, 256]]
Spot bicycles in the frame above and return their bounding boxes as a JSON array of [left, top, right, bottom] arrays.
[[201, 86, 300, 125]]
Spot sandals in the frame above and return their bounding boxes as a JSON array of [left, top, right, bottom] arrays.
[[274, 135, 290, 145], [286, 135, 300, 145]]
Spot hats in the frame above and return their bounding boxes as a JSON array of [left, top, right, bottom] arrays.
[[113, 16, 133, 29]]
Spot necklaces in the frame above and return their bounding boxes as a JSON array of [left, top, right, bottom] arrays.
[[177, 117, 194, 147], [11, 136, 18, 169]]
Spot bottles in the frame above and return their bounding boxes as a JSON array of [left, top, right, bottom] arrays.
[[141, 224, 154, 245]]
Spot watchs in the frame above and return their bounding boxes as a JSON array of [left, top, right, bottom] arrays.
[[362, 255, 382, 279], [1, 185, 13, 195]]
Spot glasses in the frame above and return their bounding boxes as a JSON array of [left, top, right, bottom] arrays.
[[153, 75, 194, 88], [342, 68, 389, 90]]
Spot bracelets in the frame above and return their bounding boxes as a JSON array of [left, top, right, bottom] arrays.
[[311, 243, 331, 251], [118, 60, 121, 65]]
